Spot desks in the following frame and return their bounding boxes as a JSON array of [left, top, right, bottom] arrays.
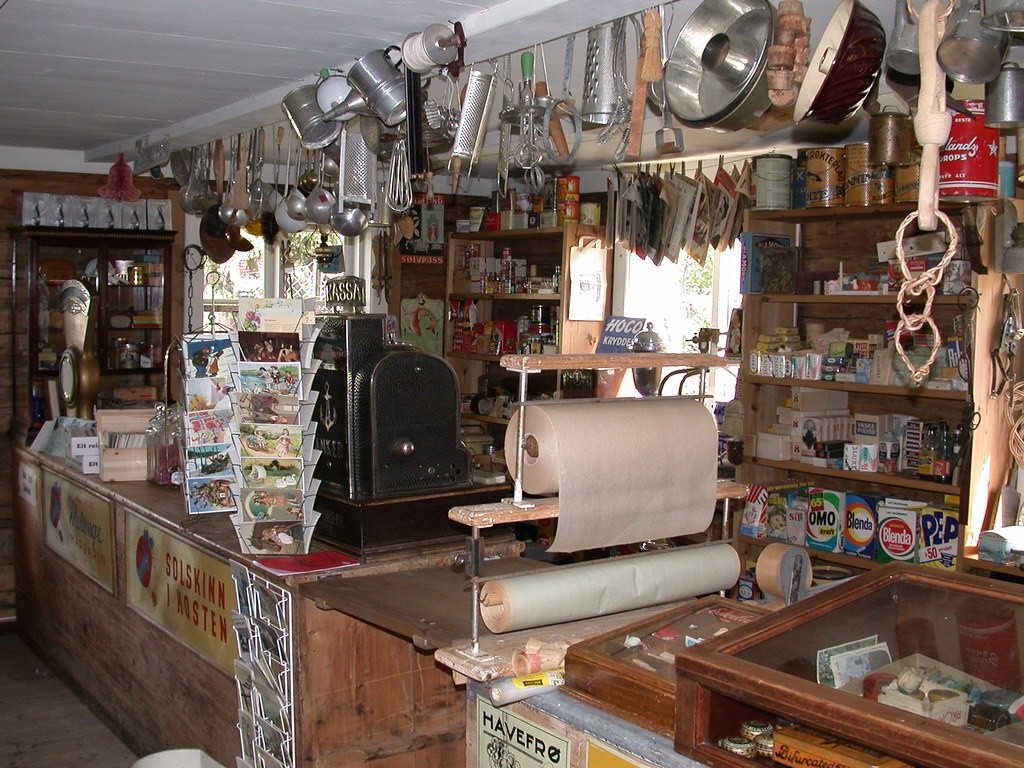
[[12, 442, 526, 767]]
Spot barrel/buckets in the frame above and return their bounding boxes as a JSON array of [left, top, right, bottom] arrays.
[[281, 85, 342, 150], [867, 104, 917, 167], [985, 63, 1024, 129]]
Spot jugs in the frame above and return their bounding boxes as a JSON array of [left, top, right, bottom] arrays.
[[323, 46, 406, 126]]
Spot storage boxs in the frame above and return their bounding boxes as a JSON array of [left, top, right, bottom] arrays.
[[740, 386, 959, 573]]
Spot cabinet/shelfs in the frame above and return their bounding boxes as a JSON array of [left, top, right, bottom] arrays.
[[443, 221, 612, 486], [4, 226, 180, 511], [732, 206, 1010, 615]]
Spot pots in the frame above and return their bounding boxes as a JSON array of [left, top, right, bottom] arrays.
[[199, 140, 240, 264], [794, 0, 887, 123], [647, 1, 777, 133]]
